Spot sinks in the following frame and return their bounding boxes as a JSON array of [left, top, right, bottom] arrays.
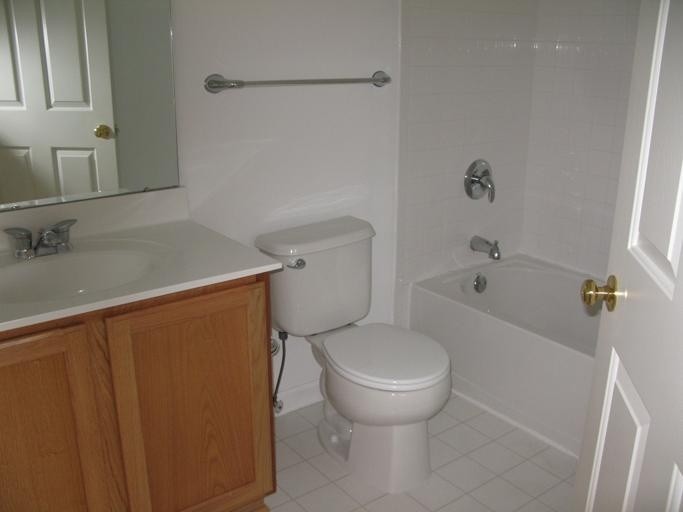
[[0, 238, 168, 302]]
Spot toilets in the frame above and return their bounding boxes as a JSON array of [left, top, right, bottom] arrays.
[[253, 215, 453, 494]]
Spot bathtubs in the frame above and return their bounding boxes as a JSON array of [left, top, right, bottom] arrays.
[[410, 252, 608, 459]]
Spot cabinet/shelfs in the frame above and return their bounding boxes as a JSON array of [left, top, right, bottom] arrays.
[[0, 271, 276, 511]]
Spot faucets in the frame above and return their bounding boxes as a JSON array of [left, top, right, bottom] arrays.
[[34, 230, 74, 256], [469, 234, 501, 261]]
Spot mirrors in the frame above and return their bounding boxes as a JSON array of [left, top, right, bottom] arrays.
[[0, 0, 179, 213]]
